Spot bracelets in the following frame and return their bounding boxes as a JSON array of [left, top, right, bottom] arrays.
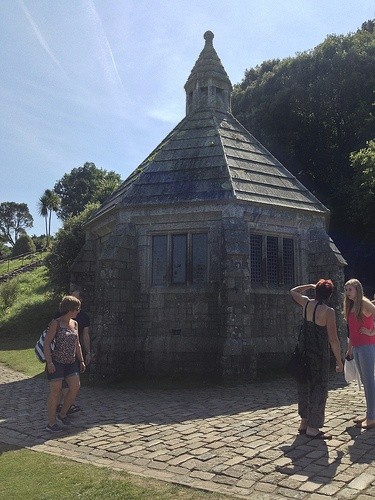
[[47, 362, 53, 365], [80, 361, 84, 362]]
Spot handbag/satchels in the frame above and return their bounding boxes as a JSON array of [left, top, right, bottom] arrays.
[[34, 319, 59, 364], [344, 354, 360, 391], [288, 347, 310, 382]]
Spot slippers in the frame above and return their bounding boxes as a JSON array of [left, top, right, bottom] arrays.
[[297, 429, 306, 433], [306, 431, 332, 439]]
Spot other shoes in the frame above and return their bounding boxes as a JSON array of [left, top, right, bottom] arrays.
[[55, 415, 72, 426], [45, 424, 63, 432], [353, 416, 375, 428]]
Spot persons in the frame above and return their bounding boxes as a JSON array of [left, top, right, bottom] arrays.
[[56, 291, 91, 413], [344, 278, 375, 429], [44, 295, 86, 432], [291, 280, 343, 440]]
